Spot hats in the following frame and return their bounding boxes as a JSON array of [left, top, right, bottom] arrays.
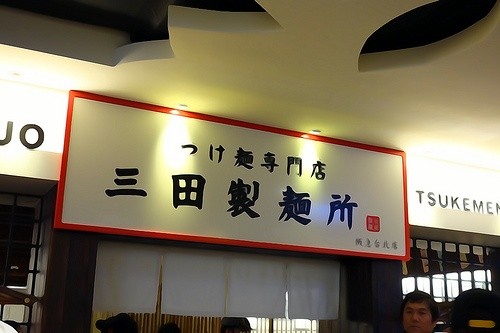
[[451, 288, 499, 329], [94, 312, 137, 332]]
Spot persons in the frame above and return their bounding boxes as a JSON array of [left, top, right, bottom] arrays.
[[158, 321, 182, 333], [400, 290, 440, 333], [446, 287, 500, 333], [1, 319, 21, 333], [218, 317, 253, 333], [94, 312, 139, 333]]
